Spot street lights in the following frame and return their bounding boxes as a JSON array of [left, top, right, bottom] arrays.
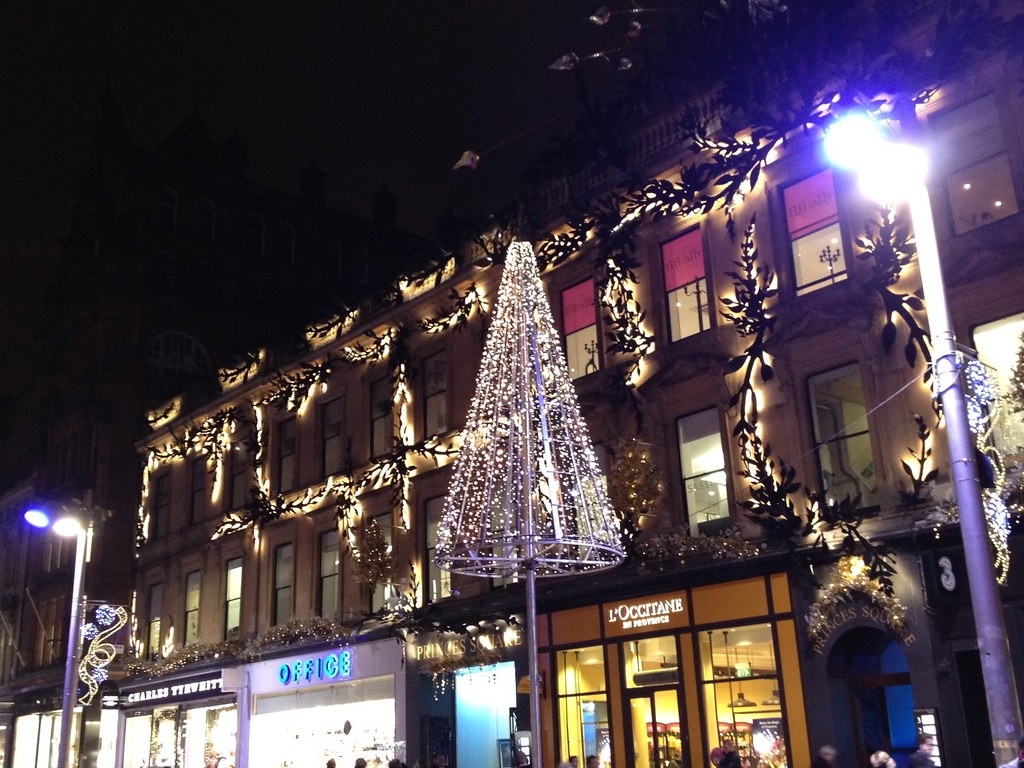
[[24, 488, 95, 768], [820, 96, 1022, 765]]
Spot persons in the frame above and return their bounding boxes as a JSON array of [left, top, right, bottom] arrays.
[[515, 750, 532, 768], [326, 752, 446, 768], [569, 755, 599, 768], [716, 739, 741, 768]]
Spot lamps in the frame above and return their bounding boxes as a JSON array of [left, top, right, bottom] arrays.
[[763, 690, 781, 706], [727, 693, 756, 707]]
